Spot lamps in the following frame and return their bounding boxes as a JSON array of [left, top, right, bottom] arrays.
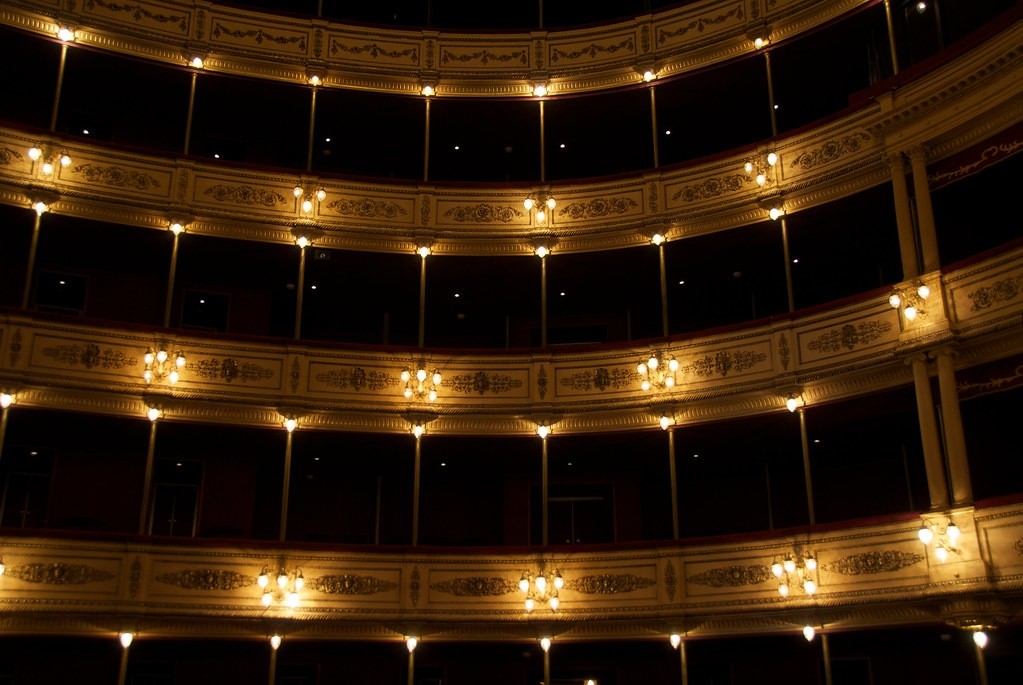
[[918, 512, 960, 563], [537, 241, 549, 257], [651, 207, 790, 251], [29, 138, 72, 174], [1, 350, 809, 439], [772, 543, 817, 601], [59, 24, 781, 98], [523, 190, 555, 223], [293, 176, 330, 213], [257, 559, 306, 610], [887, 279, 930, 321], [298, 233, 308, 247], [418, 241, 434, 258], [117, 624, 999, 650], [36, 198, 46, 216], [516, 568, 566, 615], [744, 152, 781, 186], [173, 217, 183, 236]]
